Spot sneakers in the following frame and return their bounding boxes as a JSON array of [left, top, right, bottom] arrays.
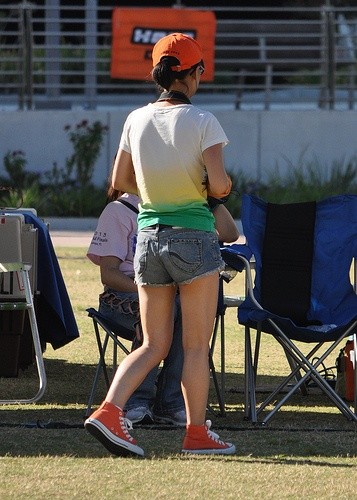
[[84, 401, 144, 457], [182, 425, 236, 455]]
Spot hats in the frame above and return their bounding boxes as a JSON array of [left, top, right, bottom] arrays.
[[152, 33, 202, 71]]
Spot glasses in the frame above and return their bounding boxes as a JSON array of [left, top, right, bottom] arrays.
[[190, 66, 205, 77]]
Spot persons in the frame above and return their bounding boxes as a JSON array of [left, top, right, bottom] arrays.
[[79, 33, 237, 455], [87, 151, 239, 427]]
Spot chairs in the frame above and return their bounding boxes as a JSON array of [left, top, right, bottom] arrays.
[[0, 206, 79, 404], [84, 192, 357, 425]]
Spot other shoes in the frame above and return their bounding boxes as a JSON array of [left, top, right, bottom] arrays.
[[153, 410, 187, 427], [125, 407, 155, 425]]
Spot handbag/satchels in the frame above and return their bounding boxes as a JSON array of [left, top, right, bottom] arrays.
[[335, 341, 357, 401]]
